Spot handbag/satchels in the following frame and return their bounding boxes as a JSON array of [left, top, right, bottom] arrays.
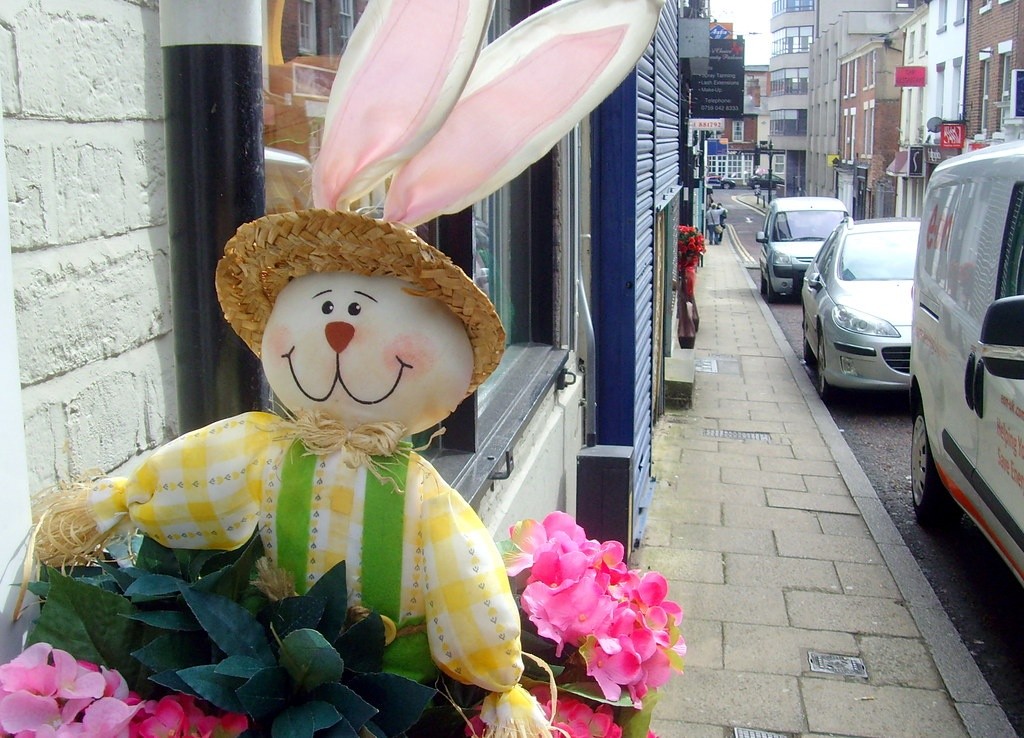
[[714, 223, 723, 234]]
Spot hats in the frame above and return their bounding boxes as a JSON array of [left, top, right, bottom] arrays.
[[710, 203, 717, 209]]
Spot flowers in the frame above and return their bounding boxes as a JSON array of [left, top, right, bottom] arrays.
[[462, 509, 686, 738], [0, 642, 247, 738], [678, 225, 707, 273]]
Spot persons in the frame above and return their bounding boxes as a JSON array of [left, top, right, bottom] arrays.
[[706, 202, 728, 245]]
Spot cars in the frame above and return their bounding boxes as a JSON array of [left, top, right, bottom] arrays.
[[721, 178, 735, 189], [748, 173, 785, 189]]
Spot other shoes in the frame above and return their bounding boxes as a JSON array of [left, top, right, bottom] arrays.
[[709, 241, 714, 245]]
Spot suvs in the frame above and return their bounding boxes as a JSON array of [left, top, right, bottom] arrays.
[[801, 215, 923, 402]]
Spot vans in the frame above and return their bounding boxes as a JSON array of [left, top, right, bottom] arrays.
[[756, 197, 852, 302], [909, 142, 1024, 590]]
